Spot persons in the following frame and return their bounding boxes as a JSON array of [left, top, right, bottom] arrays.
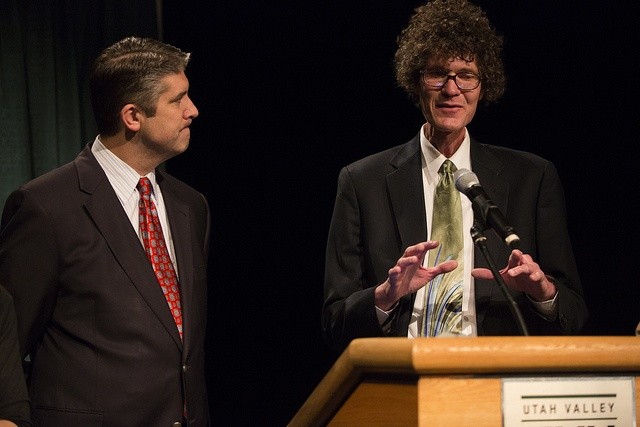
[[0, 34, 217, 427], [320, 1, 589, 344]]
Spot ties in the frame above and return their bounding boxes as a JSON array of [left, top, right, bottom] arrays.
[[419, 159, 464, 338], [135, 176, 185, 339]]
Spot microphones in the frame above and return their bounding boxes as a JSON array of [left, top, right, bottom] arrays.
[[454, 168, 521, 250]]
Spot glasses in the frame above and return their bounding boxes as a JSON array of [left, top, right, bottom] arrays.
[[419, 68, 484, 90]]
